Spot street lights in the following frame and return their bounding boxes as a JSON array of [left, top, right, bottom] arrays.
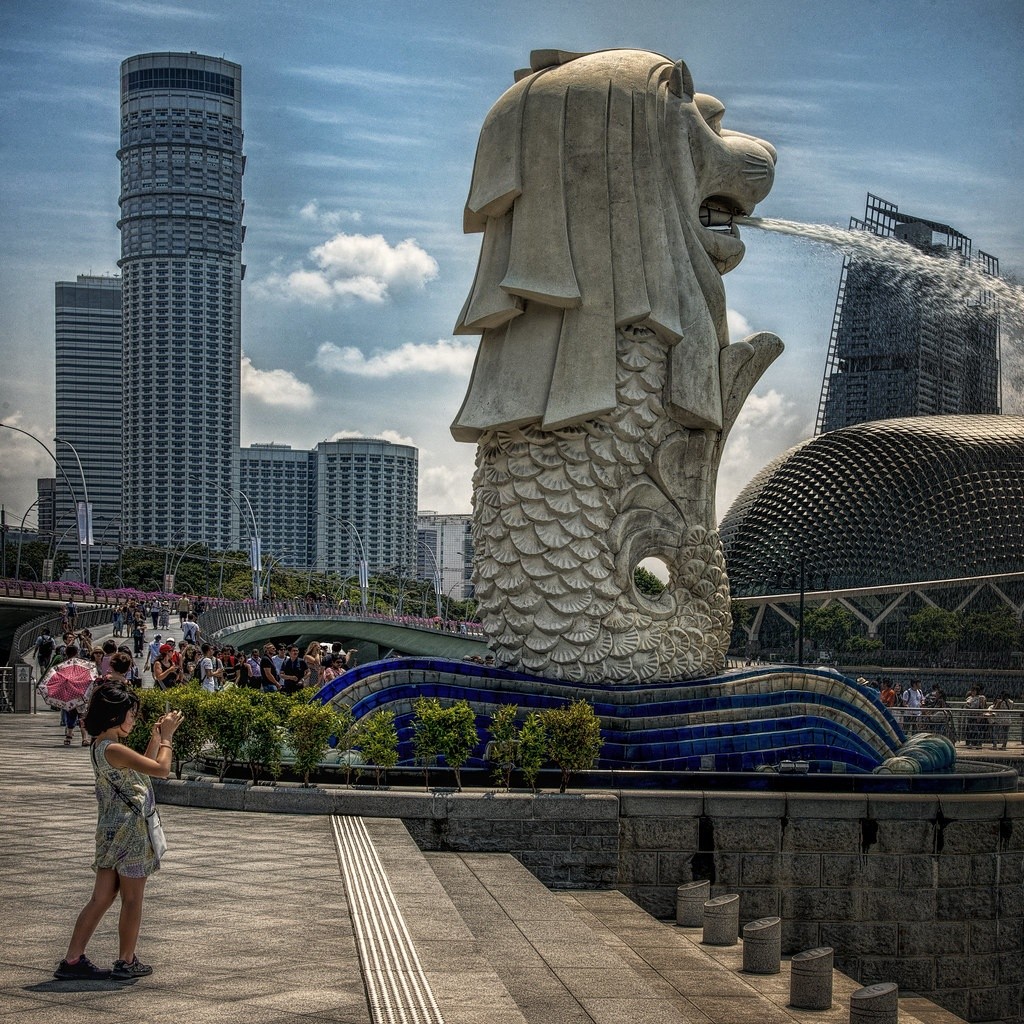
[[257, 545, 478, 625], [406, 536, 443, 618], [0, 423, 93, 586], [13, 493, 120, 589], [187, 472, 262, 611], [313, 508, 368, 610], [161, 522, 240, 598]]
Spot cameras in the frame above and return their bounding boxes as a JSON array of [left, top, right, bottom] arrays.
[[159, 723, 178, 734]]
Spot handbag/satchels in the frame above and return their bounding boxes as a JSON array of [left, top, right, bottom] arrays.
[[145, 808, 168, 859]]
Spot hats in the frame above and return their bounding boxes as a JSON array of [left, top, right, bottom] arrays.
[[159, 645, 173, 654], [162, 601, 169, 606], [166, 638, 175, 644], [182, 592, 186, 596], [142, 662, 150, 673], [90, 646, 106, 656]]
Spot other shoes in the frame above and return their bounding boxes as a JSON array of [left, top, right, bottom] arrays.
[[134, 653, 143, 658], [82, 740, 89, 746], [161, 627, 169, 630], [64, 736, 72, 746], [114, 634, 133, 638]]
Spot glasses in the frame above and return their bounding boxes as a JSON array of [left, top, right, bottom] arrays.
[[279, 648, 286, 651], [94, 651, 103, 654], [168, 650, 173, 652]]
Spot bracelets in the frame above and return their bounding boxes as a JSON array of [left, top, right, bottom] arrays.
[[159, 742, 173, 751]]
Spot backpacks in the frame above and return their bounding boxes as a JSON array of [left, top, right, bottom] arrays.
[[184, 623, 196, 644], [194, 658, 207, 685], [39, 636, 53, 656]]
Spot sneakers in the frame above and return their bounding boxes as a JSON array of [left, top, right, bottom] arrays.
[[111, 954, 153, 979], [53, 954, 111, 979]]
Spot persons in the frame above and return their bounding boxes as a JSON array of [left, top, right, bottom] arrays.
[[52, 680, 188, 981], [847, 673, 1021, 753], [32, 584, 495, 749]]
